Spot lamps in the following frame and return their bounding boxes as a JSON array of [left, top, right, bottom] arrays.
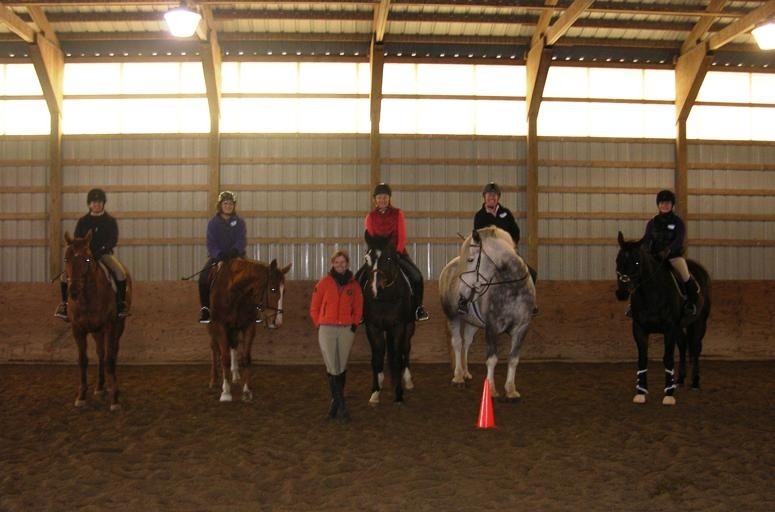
[[164, 1, 201, 38], [749, 17, 775, 51]]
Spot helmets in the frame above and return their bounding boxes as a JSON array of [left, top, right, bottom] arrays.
[[656, 189, 676, 206], [373, 183, 392, 197], [482, 182, 501, 197], [87, 188, 107, 202], [218, 191, 236, 203]]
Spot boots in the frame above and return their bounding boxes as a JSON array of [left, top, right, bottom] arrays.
[[413, 280, 428, 318], [199, 283, 211, 321], [57, 281, 68, 318], [116, 278, 128, 316], [683, 277, 698, 305], [325, 369, 346, 422], [326, 372, 353, 424]]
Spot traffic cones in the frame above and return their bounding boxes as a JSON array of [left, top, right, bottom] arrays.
[[475, 379, 497, 430]]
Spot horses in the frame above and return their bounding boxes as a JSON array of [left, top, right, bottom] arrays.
[[204, 255, 292, 402], [63, 228, 133, 412], [438, 224, 537, 403], [360, 229, 421, 407], [615, 230, 712, 406]]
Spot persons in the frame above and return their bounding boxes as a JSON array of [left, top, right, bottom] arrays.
[[197, 191, 261, 324], [353, 184, 428, 324], [309, 250, 365, 423], [53, 189, 130, 318], [623, 188, 700, 317], [453, 181, 539, 317]]
